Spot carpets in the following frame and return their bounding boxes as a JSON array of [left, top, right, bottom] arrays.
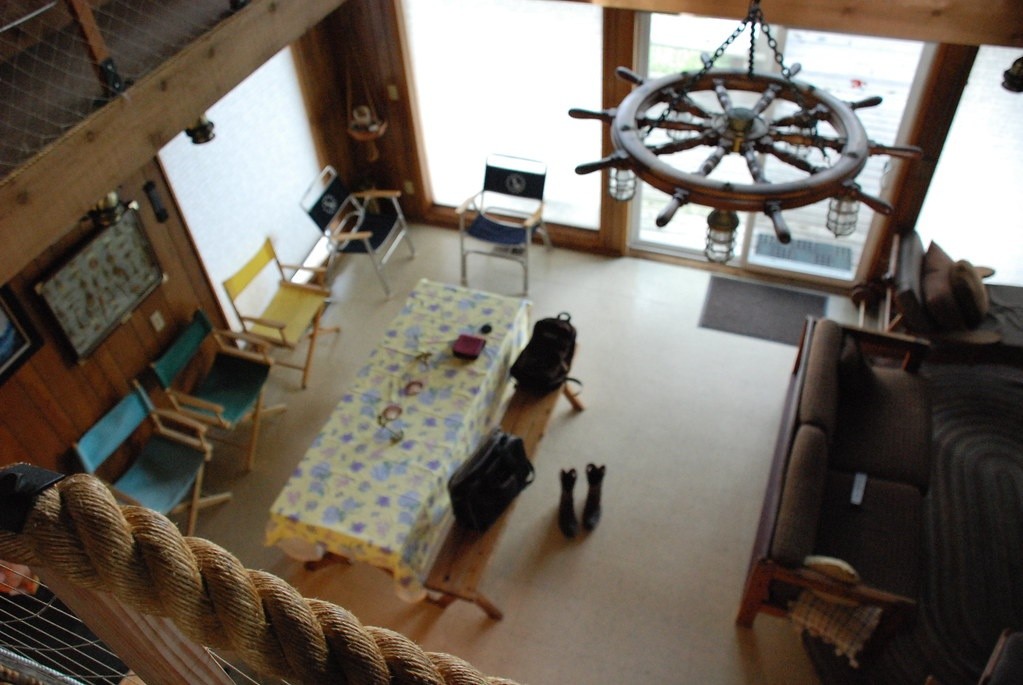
[[702, 276, 828, 346], [802, 372, 1023, 685]]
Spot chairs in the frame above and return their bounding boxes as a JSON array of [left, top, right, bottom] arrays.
[[299, 165, 418, 301], [221, 237, 342, 390], [71, 378, 234, 537], [147, 305, 288, 476], [455, 153, 552, 296]]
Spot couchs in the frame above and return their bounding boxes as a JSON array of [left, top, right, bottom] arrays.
[[735, 314, 939, 667]]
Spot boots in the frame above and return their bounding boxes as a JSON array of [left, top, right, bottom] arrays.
[[582, 463, 606, 531], [558, 468, 579, 539]]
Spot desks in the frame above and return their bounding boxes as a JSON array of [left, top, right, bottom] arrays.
[[267, 278, 539, 607]]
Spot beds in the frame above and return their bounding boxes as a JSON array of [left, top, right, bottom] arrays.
[[882, 227, 1023, 373]]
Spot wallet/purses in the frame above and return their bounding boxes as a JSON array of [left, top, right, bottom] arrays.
[[453, 334, 486, 360]]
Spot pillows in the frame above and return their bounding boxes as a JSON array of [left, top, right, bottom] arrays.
[[950, 259, 990, 326], [923, 241, 958, 331], [836, 331, 878, 408]]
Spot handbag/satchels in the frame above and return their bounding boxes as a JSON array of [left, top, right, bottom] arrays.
[[448, 428, 534, 536]]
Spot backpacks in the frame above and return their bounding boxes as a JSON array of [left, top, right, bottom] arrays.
[[509, 312, 583, 398]]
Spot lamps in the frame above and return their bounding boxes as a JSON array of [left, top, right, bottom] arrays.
[[570, 0, 926, 267], [185, 113, 215, 145], [87, 190, 123, 228]]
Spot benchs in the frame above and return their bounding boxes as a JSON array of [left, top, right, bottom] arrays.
[[422, 343, 584, 621]]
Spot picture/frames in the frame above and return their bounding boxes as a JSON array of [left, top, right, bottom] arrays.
[[24, 201, 169, 370], [0, 282, 46, 389]]
